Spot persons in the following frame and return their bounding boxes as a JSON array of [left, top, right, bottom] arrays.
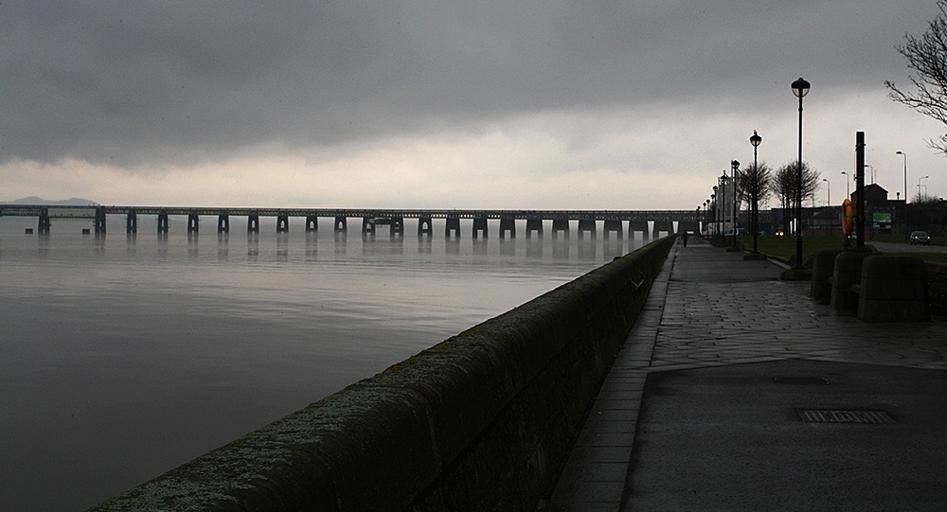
[[681, 228, 690, 247]]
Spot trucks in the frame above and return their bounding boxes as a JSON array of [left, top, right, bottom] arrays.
[[701, 222, 739, 239]]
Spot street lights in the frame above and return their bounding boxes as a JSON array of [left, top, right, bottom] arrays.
[[808, 143, 930, 240], [696, 185, 718, 243], [726, 159, 745, 253], [719, 174, 730, 250], [745, 129, 767, 261], [788, 73, 811, 279]]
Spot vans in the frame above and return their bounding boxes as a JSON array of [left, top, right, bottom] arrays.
[[723, 227, 745, 237]]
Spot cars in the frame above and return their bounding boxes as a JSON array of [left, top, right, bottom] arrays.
[[909, 229, 931, 246], [774, 230, 784, 238]]
